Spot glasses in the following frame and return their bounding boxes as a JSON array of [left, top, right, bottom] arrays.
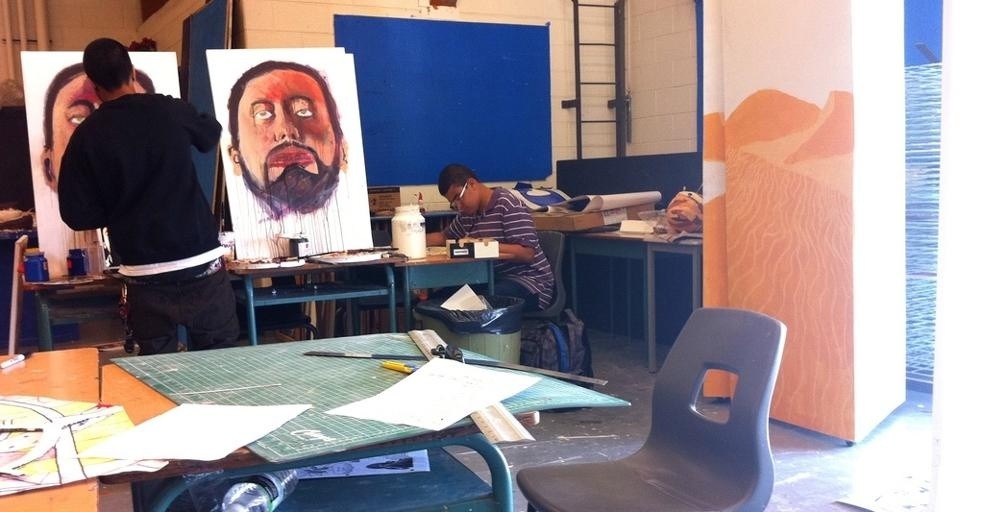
[[449, 180, 468, 211]]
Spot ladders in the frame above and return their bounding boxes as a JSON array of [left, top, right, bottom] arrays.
[[573, 1, 625, 159]]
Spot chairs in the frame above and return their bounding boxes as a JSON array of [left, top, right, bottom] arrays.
[[522, 230, 567, 317], [515, 307, 788, 511]]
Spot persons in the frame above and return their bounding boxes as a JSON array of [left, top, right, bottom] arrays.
[[58, 38, 242, 356], [426, 163, 555, 312]]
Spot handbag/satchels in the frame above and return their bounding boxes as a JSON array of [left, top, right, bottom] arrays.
[[520, 308, 594, 414]]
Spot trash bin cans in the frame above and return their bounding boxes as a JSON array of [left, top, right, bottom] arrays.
[[411, 296, 524, 364]]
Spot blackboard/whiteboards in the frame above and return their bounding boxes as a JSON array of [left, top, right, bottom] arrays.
[[332, 15, 552, 188]]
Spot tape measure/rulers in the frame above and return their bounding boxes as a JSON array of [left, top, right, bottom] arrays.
[[303, 329, 607, 444]]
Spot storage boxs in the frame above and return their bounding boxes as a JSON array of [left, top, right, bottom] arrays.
[[367, 187, 401, 217], [528, 202, 656, 232]]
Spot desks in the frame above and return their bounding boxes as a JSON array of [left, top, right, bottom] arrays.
[[569, 230, 705, 374], [99, 358, 541, 511], [26, 273, 188, 352], [0, 349, 104, 511], [227, 243, 495, 346]]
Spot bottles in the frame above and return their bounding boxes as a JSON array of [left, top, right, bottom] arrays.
[[221, 468, 298, 512], [277, 232, 310, 262], [66, 241, 103, 276], [23, 251, 49, 281]]
